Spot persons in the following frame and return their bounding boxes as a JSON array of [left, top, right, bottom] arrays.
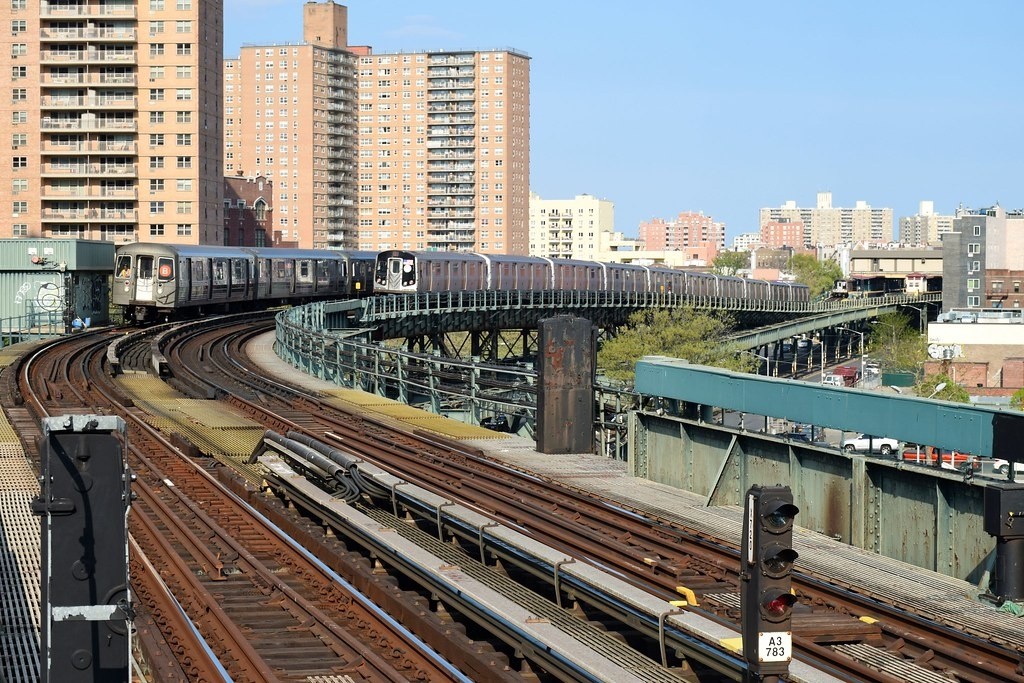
[[118, 264, 130, 278]]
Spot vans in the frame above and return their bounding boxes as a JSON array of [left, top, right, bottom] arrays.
[[823, 375, 846, 387]]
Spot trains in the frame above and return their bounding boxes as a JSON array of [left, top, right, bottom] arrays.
[[110, 242, 379, 326], [373, 249, 810, 304]]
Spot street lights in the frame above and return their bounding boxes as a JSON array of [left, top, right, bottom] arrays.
[[837, 327, 864, 381], [793, 335, 824, 385], [919, 301, 938, 316], [901, 304, 922, 335], [871, 321, 896, 345], [735, 349, 767, 434]]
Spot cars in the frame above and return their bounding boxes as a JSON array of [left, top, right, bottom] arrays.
[[800, 425, 824, 443], [843, 434, 900, 455], [993, 458, 1024, 475], [902, 446, 981, 471]]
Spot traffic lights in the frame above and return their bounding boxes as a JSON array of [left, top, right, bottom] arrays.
[[756, 492, 799, 623]]
